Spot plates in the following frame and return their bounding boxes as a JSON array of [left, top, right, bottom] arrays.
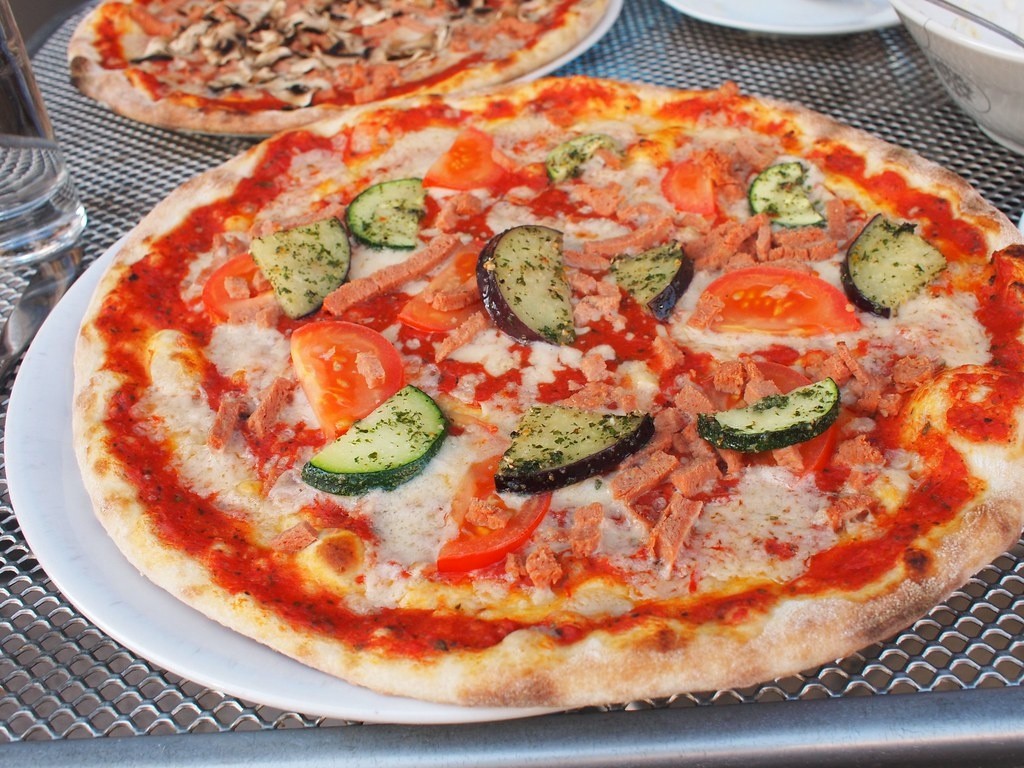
[[174, 0, 623, 137], [662, 0, 900, 36], [5, 226, 568, 723]]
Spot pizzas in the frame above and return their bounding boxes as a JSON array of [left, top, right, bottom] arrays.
[[72, 74, 1023, 710], [69, 0, 608, 137]]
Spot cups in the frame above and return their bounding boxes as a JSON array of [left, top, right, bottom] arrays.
[[0, 0, 87, 266]]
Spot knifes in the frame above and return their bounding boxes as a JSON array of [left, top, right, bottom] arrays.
[[0, 248, 82, 397]]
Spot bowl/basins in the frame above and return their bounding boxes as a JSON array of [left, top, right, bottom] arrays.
[[889, 0, 1024, 157]]
